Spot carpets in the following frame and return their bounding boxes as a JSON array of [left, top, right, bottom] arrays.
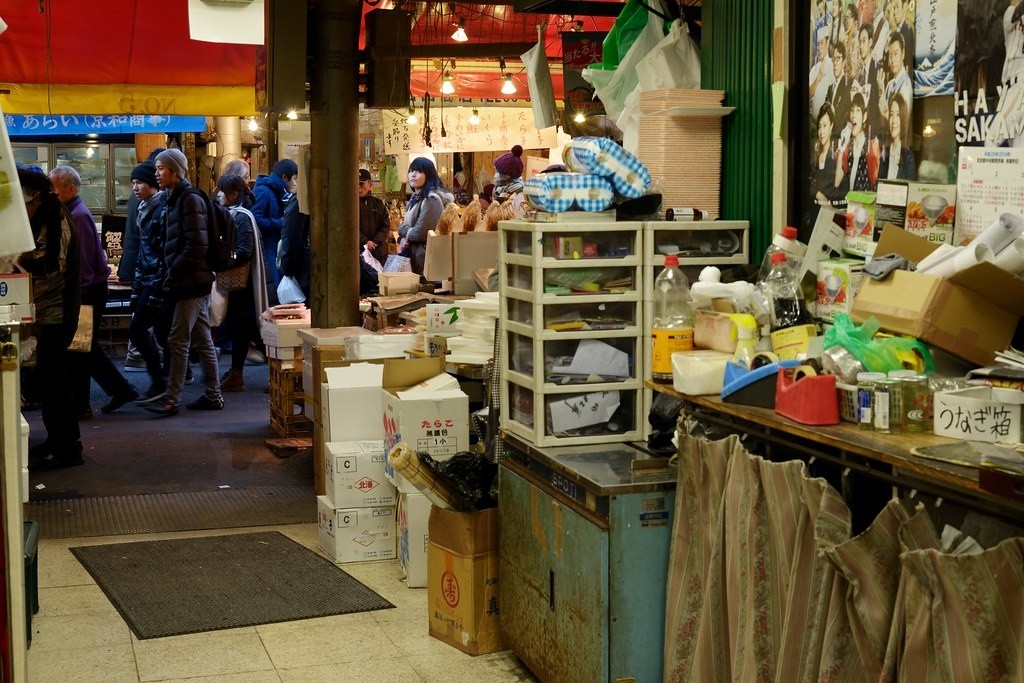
[[68, 530, 397, 640]]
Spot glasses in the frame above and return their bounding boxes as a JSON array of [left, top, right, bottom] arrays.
[[832, 56, 842, 61]]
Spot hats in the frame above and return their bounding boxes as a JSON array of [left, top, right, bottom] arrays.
[[359, 169, 371, 183], [817, 26, 829, 41], [494, 145, 523, 178], [130, 160, 160, 187], [155, 148, 188, 179]]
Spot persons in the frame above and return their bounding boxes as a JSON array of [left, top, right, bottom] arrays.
[[359, 168, 390, 268], [809, 0, 913, 206], [492, 145, 524, 199], [15, 161, 140, 471], [117, 147, 311, 416], [479, 184, 496, 209], [397, 157, 444, 274]]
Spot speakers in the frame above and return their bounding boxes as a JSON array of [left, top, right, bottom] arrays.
[[364, 9, 411, 108]]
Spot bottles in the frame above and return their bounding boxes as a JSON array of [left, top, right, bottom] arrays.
[[757, 226, 808, 299], [666, 207, 708, 221], [755, 327, 772, 351], [650, 256, 694, 384], [766, 253, 809, 333]]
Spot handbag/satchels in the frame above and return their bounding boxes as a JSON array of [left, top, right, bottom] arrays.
[[377, 227, 412, 286], [277, 275, 306, 305], [209, 272, 225, 326], [67, 304, 93, 352], [362, 245, 383, 273], [217, 264, 248, 289]]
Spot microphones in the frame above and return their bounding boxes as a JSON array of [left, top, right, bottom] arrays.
[[836, 122, 852, 153]]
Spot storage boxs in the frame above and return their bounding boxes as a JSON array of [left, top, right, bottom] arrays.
[[670, 145, 1024, 446], [0, 273, 36, 324], [254, 229, 514, 655]]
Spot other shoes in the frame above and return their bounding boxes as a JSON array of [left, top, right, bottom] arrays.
[[220, 369, 245, 391], [186, 391, 224, 409], [30, 436, 85, 470], [146, 395, 181, 414], [135, 382, 167, 403], [101, 379, 140, 413], [185, 369, 194, 384]]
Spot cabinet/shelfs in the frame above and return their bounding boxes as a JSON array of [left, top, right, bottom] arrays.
[[496, 219, 751, 450]]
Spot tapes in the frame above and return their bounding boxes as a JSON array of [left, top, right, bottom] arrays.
[[791, 364, 816, 383], [823, 346, 863, 384], [749, 351, 777, 373]]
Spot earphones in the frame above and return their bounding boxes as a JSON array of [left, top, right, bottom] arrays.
[[174, 172, 176, 176]]
